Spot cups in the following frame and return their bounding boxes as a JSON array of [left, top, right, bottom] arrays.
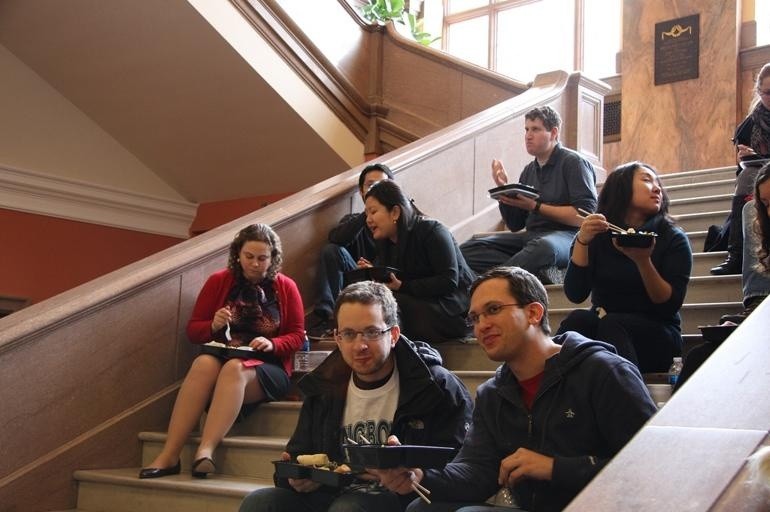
[[647, 383, 672, 406]]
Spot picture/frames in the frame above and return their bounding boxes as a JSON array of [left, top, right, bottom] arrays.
[[653, 12, 700, 87], [0, 295, 33, 319]]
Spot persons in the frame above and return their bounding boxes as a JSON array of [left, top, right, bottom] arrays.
[[365, 266, 659, 512], [358, 179, 481, 343], [239, 281, 474, 512], [459, 107, 599, 284], [555, 162, 693, 373], [139, 225, 303, 478], [670, 161, 770, 395], [710, 63, 770, 275], [307, 164, 393, 340]]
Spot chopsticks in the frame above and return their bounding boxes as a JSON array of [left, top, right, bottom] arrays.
[[345, 434, 433, 507], [575, 207, 627, 233]]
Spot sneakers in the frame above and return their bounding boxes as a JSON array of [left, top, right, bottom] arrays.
[[538, 265, 570, 287], [720, 297, 764, 324], [710, 252, 742, 274], [308, 319, 339, 341]]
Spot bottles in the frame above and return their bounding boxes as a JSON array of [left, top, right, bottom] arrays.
[[495, 484, 523, 512], [668, 357, 684, 387], [293, 330, 311, 371]]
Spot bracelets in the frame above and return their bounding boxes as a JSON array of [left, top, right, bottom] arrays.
[[576, 235, 593, 246]]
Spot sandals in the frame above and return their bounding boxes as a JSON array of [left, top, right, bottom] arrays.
[[139, 456, 182, 478], [190, 457, 216, 477]]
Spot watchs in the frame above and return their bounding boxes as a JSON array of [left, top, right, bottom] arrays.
[[531, 200, 542, 216]]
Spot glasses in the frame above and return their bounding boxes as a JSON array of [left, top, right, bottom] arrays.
[[334, 323, 394, 343], [461, 301, 529, 328]]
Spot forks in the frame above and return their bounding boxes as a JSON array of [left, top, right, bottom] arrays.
[[224, 321, 233, 342]]
